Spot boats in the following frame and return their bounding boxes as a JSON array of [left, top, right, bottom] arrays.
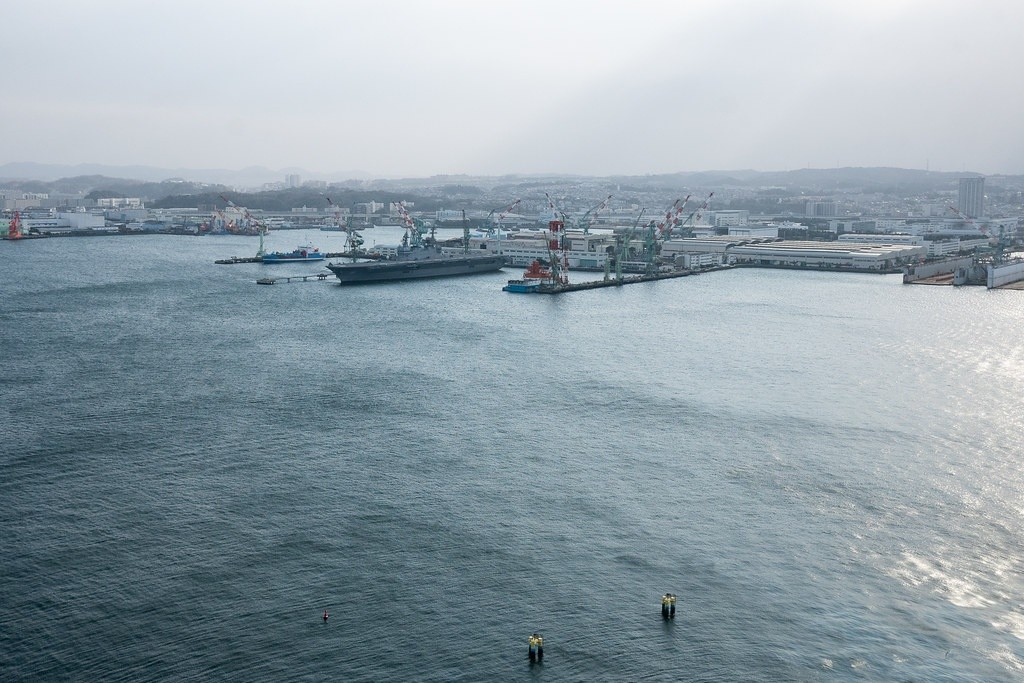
[[262, 236, 326, 263], [324, 218, 514, 284]]
